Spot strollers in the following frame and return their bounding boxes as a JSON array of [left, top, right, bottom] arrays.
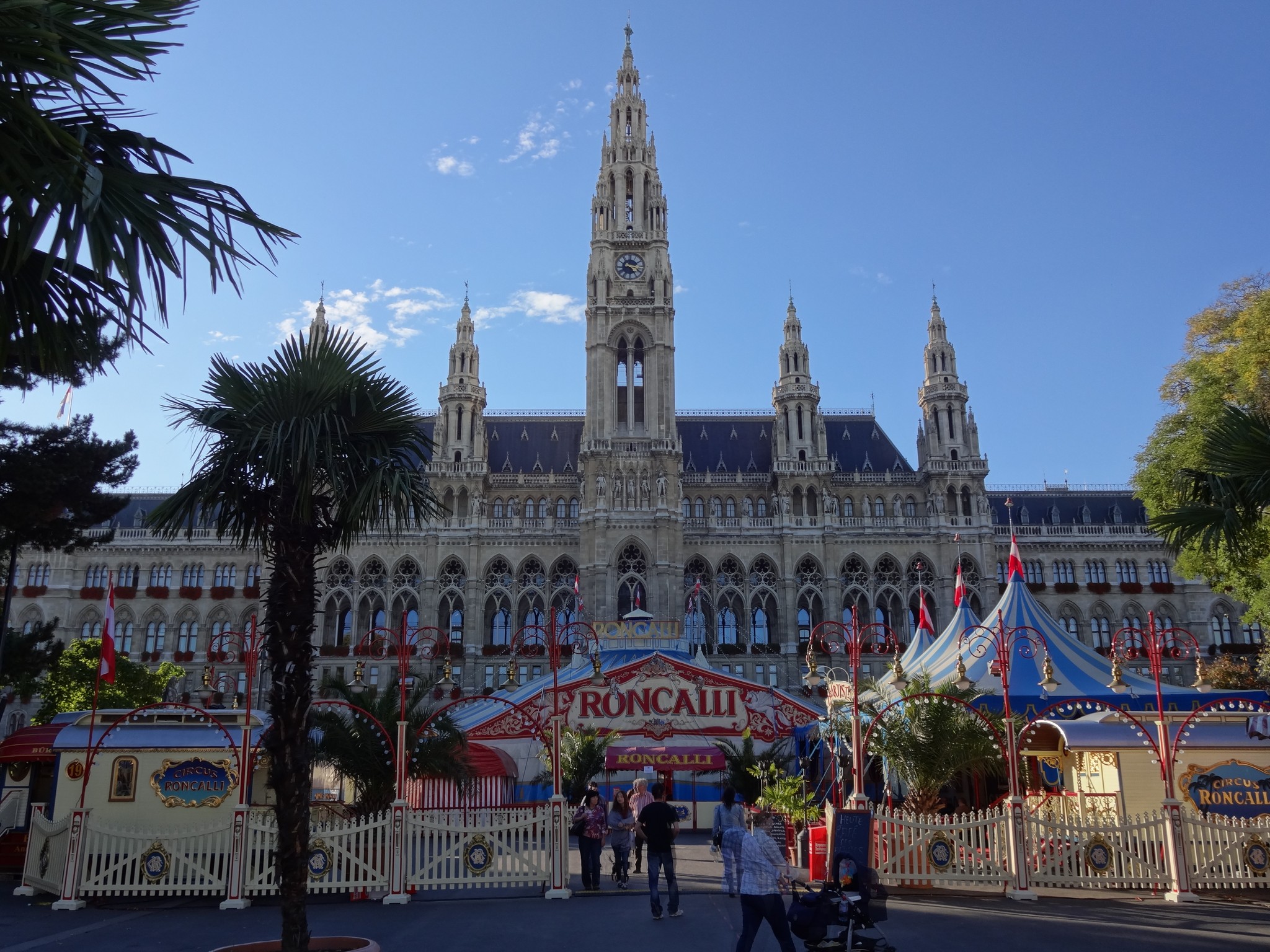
[[787, 851, 899, 952]]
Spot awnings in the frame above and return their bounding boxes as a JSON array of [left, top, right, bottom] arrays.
[[0, 721, 69, 766]]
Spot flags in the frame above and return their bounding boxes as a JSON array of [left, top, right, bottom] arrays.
[[919, 588, 935, 636], [99, 581, 116, 686], [578, 595, 584, 612], [574, 575, 579, 595], [635, 588, 640, 608], [694, 575, 700, 597], [954, 564, 967, 608], [688, 594, 694, 614], [1008, 531, 1024, 583]]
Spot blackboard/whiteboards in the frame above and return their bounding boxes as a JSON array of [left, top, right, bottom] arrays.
[[825, 809, 876, 893], [749, 812, 790, 867]]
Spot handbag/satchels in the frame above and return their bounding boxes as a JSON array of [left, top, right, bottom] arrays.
[[709, 834, 722, 857], [786, 900, 817, 926], [570, 819, 585, 834]]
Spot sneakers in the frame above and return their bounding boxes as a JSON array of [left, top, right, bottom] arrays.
[[585, 885, 592, 890], [729, 892, 736, 898], [668, 908, 684, 917], [620, 882, 628, 890], [616, 880, 622, 888], [653, 913, 664, 920], [593, 884, 600, 890]]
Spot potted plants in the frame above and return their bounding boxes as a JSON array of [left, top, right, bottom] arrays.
[[144, 315, 455, 952]]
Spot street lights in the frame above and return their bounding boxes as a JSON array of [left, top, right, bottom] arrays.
[[193, 612, 268, 911], [346, 608, 458, 904], [954, 608, 1064, 901], [498, 606, 602, 897], [797, 607, 912, 906], [1106, 611, 1215, 905]]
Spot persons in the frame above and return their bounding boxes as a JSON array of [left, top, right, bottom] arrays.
[[608, 790, 636, 891], [628, 778, 655, 874], [468, 492, 553, 516], [770, 490, 838, 515], [579, 472, 684, 501], [633, 782, 684, 919], [572, 789, 607, 892], [626, 788, 637, 854], [738, 805, 808, 951], [580, 781, 609, 836]]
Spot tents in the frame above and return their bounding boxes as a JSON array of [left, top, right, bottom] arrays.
[[819, 620, 936, 732], [405, 742, 520, 837], [413, 607, 833, 836], [834, 596, 986, 729], [859, 568, 1270, 801], [794, 717, 885, 810], [606, 744, 727, 833]]
